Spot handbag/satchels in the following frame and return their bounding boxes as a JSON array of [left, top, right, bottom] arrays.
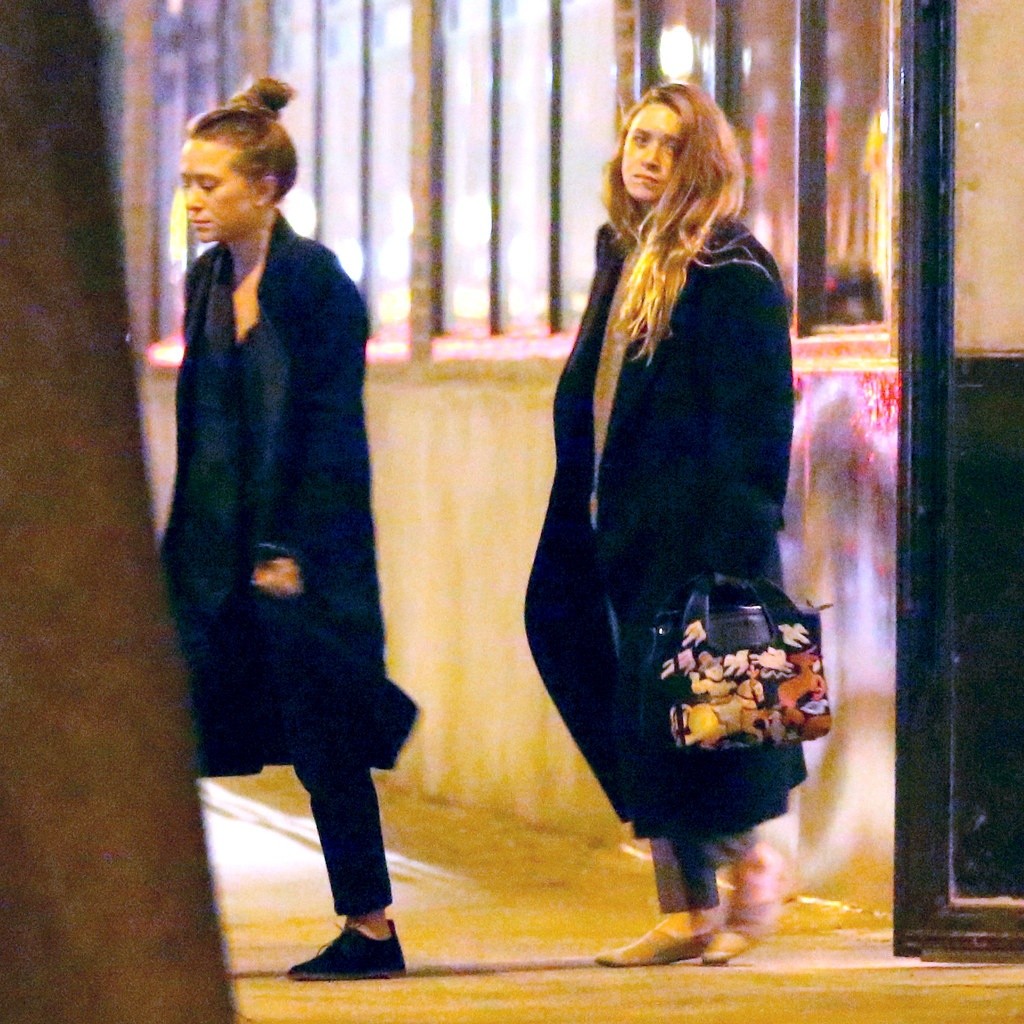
[[637, 570, 832, 752]]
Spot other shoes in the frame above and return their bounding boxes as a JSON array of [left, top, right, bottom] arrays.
[[288, 917, 405, 983], [597, 918, 724, 967], [703, 850, 789, 964]]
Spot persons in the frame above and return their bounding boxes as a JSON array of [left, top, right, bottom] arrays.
[[523, 82, 810, 968], [144, 78, 420, 982]]
